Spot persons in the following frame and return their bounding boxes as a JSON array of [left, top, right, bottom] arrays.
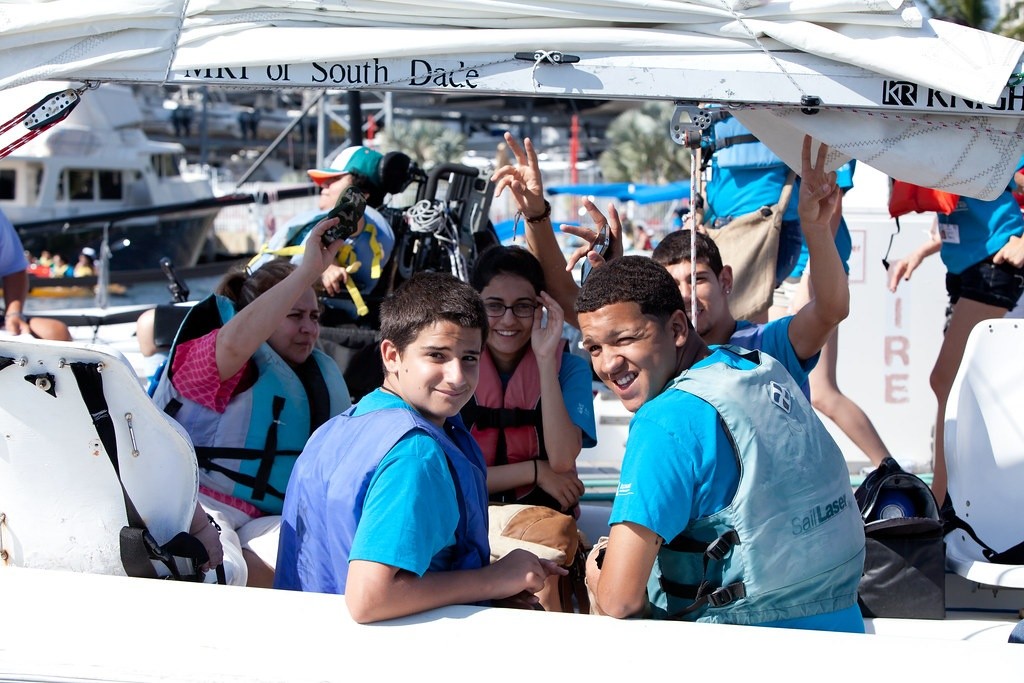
[[560, 196, 868, 634], [178, 104, 330, 145], [272, 270, 570, 624], [23, 249, 94, 278], [136, 145, 396, 358], [147, 217, 353, 588], [458, 245, 598, 521], [0, 208, 73, 343], [488, 131, 851, 406], [889, 151, 1024, 511], [681, 100, 893, 465], [616, 204, 689, 252]]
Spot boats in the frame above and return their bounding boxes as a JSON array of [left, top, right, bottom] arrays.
[[176, 162, 332, 258], [0, 338, 1024, 682], [0, 81, 257, 278], [0, 280, 128, 297]]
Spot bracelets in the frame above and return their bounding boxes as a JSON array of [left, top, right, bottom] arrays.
[[531, 459, 538, 486], [512, 199, 551, 241], [682, 213, 691, 222], [5, 311, 26, 322]]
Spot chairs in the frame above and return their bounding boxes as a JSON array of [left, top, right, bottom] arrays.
[[944, 317, 1023, 590], [0, 338, 199, 581]]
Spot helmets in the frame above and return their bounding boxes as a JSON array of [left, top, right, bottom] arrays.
[[853, 456, 943, 540]]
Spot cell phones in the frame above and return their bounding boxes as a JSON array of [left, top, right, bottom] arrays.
[[581, 223, 610, 286]]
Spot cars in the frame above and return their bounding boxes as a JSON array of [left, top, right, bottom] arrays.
[[166, 87, 393, 147]]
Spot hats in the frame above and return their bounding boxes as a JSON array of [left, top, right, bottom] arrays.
[[307, 145, 383, 188]]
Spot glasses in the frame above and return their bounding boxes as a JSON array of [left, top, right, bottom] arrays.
[[482, 302, 539, 319]]
